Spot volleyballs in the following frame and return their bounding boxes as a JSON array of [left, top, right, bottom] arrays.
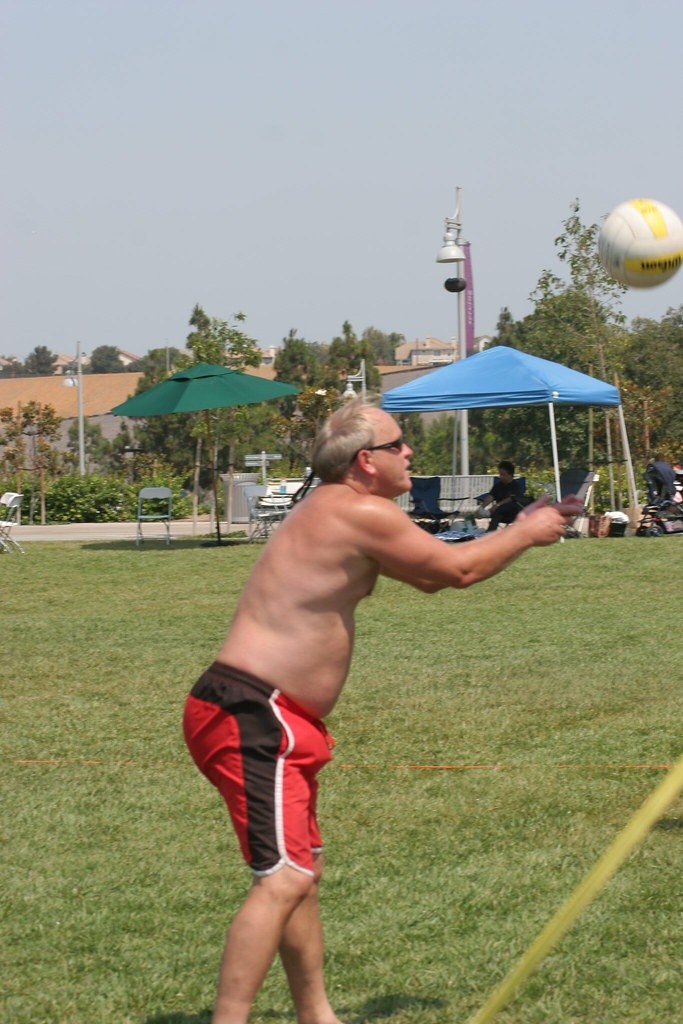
[[598, 197, 683, 289]]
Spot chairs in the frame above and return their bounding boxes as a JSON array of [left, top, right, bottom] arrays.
[[246, 471, 315, 544], [410, 476, 527, 535], [136, 487, 172, 547], [0, 492, 26, 556]]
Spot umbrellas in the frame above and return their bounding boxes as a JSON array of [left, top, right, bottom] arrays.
[[110, 361, 304, 517]]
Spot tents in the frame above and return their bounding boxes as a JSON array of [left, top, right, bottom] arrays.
[[378, 347, 639, 543]]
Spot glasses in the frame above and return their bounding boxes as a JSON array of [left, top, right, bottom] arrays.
[[349, 436, 404, 466]]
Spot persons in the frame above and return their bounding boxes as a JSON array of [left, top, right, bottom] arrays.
[[465, 462, 519, 532], [182, 390, 566, 1024], [642, 452, 679, 504]]
